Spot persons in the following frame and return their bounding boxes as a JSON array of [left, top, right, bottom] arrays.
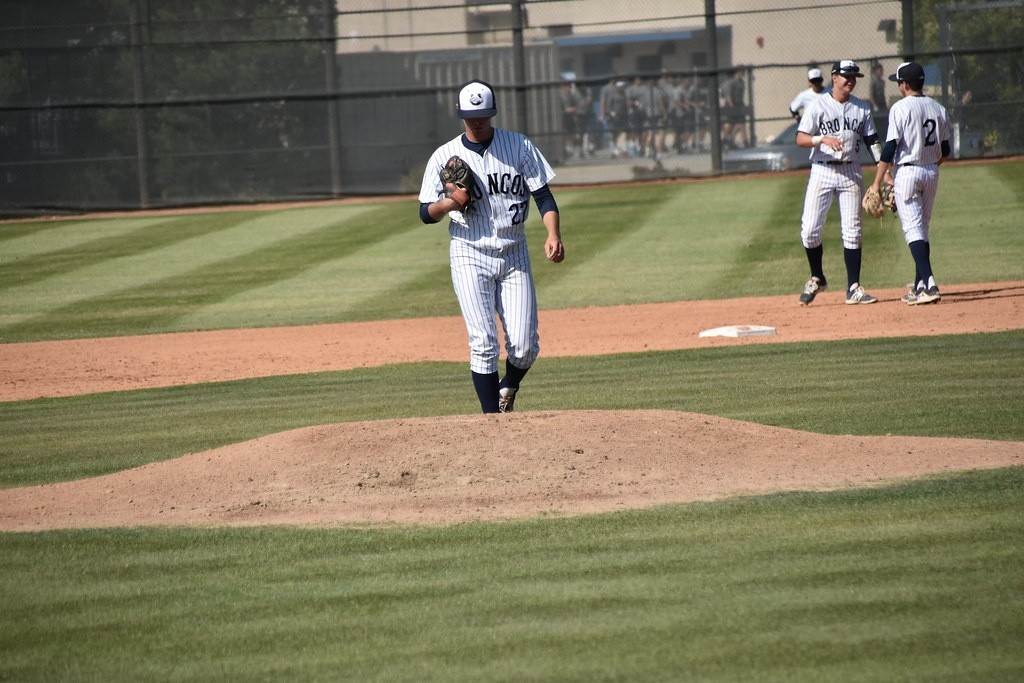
[[795, 60, 892, 305], [559, 62, 748, 159], [790, 69, 828, 124], [948, 89, 973, 123], [869, 64, 887, 112], [862, 61, 951, 305], [418, 81, 564, 413]]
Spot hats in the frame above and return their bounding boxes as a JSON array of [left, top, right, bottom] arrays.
[[888, 62, 924, 83], [831, 60, 864, 77], [808, 69, 821, 80], [456, 80, 496, 119]]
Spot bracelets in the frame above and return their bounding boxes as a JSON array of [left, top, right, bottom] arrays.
[[812, 136, 824, 144]]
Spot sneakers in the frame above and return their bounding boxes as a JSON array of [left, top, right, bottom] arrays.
[[498, 380, 519, 413], [798, 275, 827, 307], [900, 283, 941, 306], [846, 283, 878, 305]]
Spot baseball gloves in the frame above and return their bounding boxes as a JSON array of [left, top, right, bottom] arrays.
[[880, 181, 898, 214], [440, 156, 475, 213], [861, 186, 885, 218]]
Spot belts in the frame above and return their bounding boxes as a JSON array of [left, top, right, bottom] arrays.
[[904, 162, 937, 166], [818, 161, 852, 165]]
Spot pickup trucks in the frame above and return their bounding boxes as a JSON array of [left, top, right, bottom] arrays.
[[720, 106, 984, 174]]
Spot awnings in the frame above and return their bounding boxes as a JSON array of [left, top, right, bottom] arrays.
[[554, 25, 731, 48]]
